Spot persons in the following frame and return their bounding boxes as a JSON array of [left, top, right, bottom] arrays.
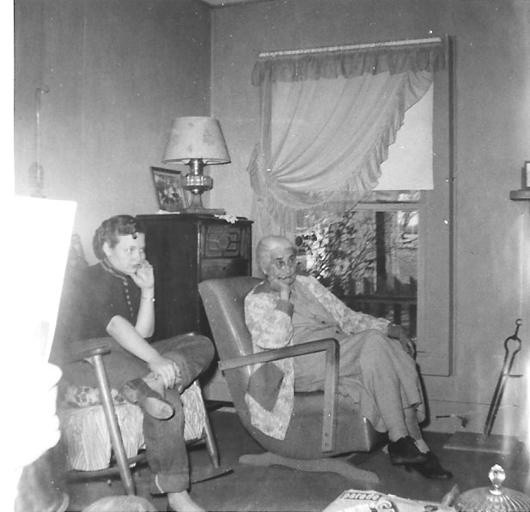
[[243, 232, 450, 481], [50, 214, 216, 512]]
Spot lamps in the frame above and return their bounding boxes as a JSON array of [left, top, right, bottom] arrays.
[[161, 116, 233, 216]]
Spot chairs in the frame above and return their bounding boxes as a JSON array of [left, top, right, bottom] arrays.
[[195, 277, 389, 486], [55, 234, 235, 503]]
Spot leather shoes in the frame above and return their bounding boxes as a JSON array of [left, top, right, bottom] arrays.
[[387, 434, 429, 466], [402, 455, 455, 482]]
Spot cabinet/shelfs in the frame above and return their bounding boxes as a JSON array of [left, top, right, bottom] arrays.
[[135, 213, 255, 338]]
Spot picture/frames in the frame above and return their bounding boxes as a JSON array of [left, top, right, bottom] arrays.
[[151, 166, 191, 213]]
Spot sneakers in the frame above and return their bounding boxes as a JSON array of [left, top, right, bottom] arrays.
[[120, 378, 175, 421]]
